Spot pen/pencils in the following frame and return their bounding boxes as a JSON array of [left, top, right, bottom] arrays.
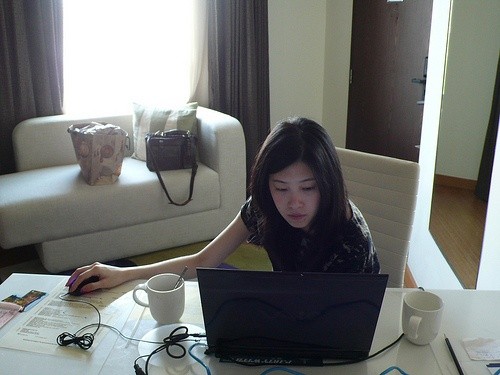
[[443, 332, 464, 375]]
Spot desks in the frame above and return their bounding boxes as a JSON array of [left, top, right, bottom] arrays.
[[0, 271, 500, 375]]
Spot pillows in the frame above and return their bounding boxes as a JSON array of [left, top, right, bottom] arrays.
[[130, 101, 198, 161]]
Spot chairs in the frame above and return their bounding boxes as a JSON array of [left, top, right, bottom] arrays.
[[334, 147, 420, 289]]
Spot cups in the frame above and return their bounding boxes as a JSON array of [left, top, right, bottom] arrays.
[[133, 273, 185, 324], [402, 291, 444, 345]]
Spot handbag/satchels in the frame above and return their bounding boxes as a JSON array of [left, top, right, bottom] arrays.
[[67, 121, 130, 186], [145, 129, 200, 206]]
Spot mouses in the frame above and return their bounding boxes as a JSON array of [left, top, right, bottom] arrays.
[[70, 275, 99, 296]]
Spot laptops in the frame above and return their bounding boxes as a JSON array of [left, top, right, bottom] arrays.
[[196, 267, 389, 362]]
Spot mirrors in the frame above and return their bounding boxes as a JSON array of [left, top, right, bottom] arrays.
[[428, 0, 500, 290]]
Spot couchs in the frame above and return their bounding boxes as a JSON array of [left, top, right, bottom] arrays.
[[0, 104, 247, 275]]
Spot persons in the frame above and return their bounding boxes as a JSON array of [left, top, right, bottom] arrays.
[[66, 117, 381, 296]]
